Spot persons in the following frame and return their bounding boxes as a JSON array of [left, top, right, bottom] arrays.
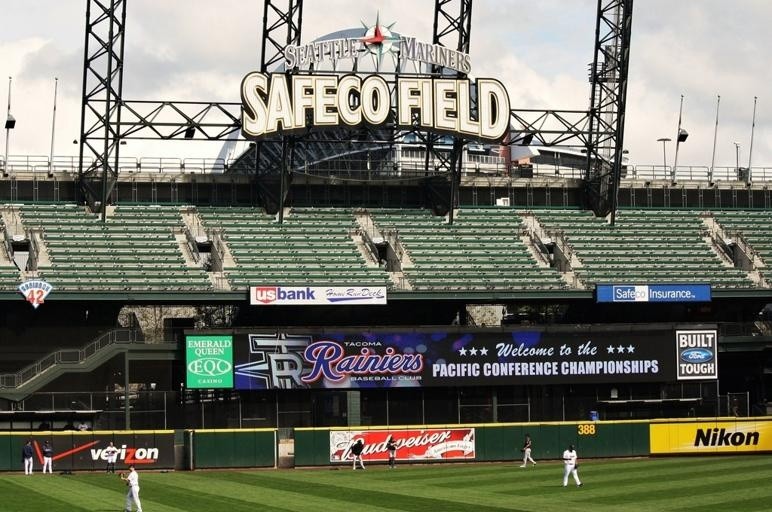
[[38, 420, 50, 431], [387, 435, 397, 469], [688, 406, 696, 418], [351, 439, 366, 471], [120, 463, 143, 512], [730, 403, 740, 417], [63, 421, 83, 431], [561, 444, 584, 488], [519, 433, 537, 468], [78, 420, 88, 431], [105, 442, 118, 474], [21, 439, 35, 476]]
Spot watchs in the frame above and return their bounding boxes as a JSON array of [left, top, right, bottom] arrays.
[[41, 438, 54, 475]]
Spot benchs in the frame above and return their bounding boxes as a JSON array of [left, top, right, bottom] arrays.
[[0, 199, 772, 293]]
